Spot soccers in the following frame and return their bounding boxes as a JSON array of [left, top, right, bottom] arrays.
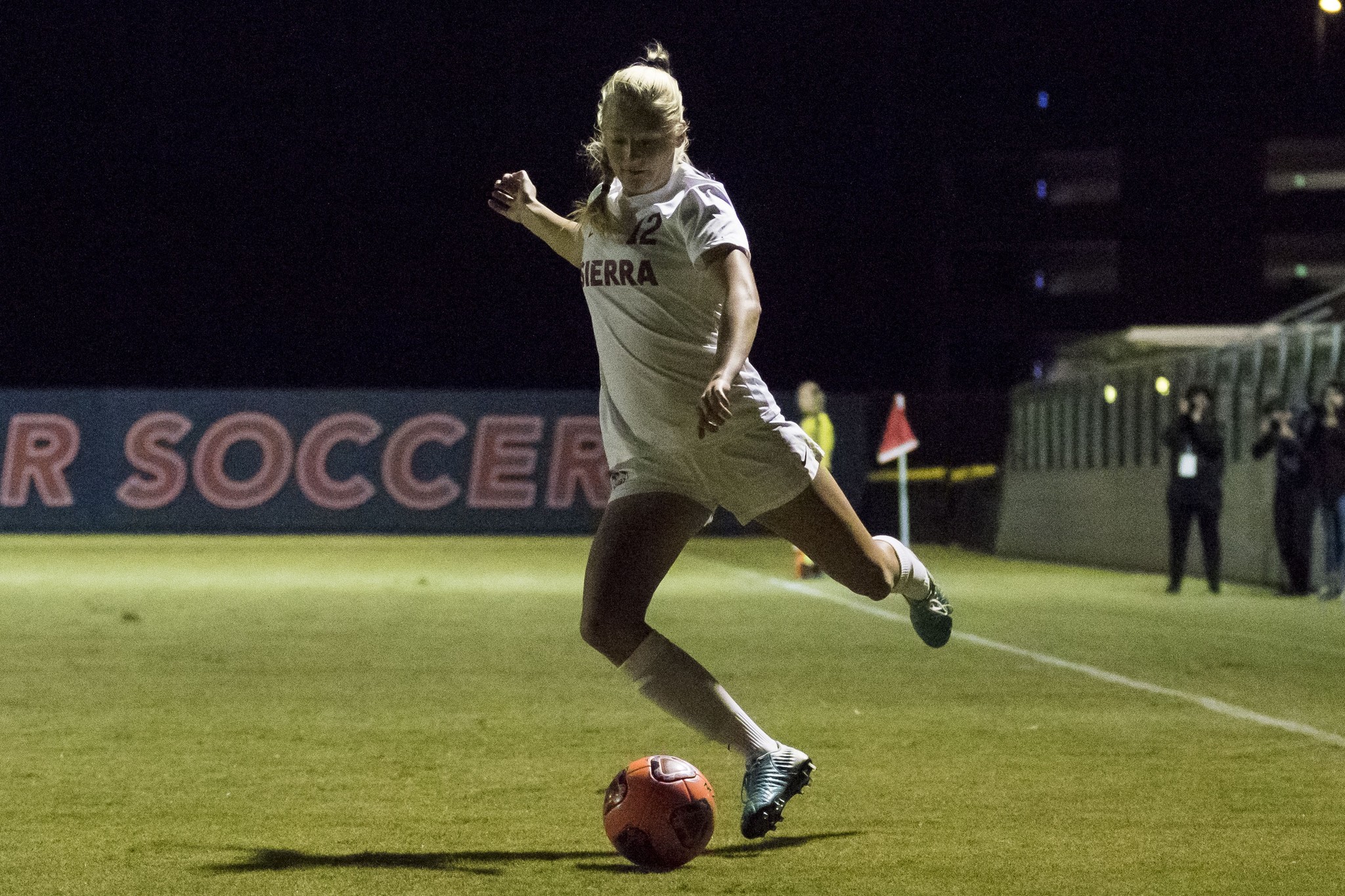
[[604, 754, 716, 873]]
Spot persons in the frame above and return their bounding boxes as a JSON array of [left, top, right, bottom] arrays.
[[1249, 397, 1326, 597], [486, 40, 952, 842], [1158, 385, 1223, 597], [793, 381, 838, 579], [1310, 381, 1345, 599]]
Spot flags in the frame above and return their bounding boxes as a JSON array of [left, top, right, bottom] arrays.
[[876, 399, 919, 466]]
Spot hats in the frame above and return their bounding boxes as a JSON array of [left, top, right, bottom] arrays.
[[1262, 394, 1287, 413], [1185, 384, 1214, 407]]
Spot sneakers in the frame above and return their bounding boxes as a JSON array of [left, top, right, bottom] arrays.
[[741, 739, 818, 840], [901, 546, 954, 648]]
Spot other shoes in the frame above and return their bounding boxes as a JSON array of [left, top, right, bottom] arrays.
[[1210, 583, 1219, 594], [1166, 582, 1180, 593]]
